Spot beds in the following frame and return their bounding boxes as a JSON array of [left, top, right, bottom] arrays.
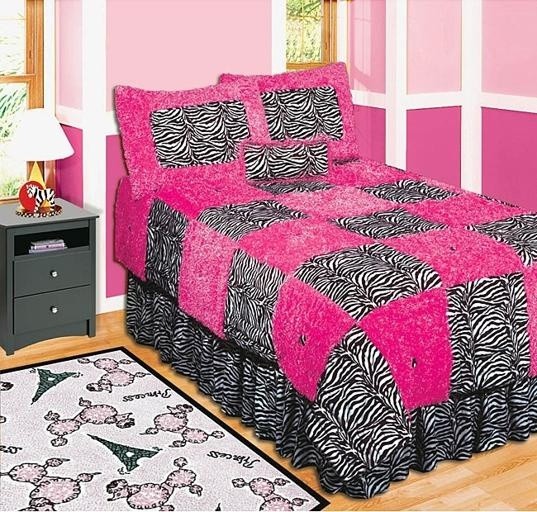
[[114, 158, 537, 499]]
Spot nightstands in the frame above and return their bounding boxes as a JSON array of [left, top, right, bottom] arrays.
[[0, 197, 101, 357]]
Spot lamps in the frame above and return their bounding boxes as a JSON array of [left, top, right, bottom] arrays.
[[9, 107, 74, 218]]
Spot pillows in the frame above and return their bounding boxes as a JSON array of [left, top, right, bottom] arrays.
[[112, 60, 361, 201]]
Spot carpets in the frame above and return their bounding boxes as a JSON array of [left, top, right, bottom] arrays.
[[0, 345, 331, 512]]
